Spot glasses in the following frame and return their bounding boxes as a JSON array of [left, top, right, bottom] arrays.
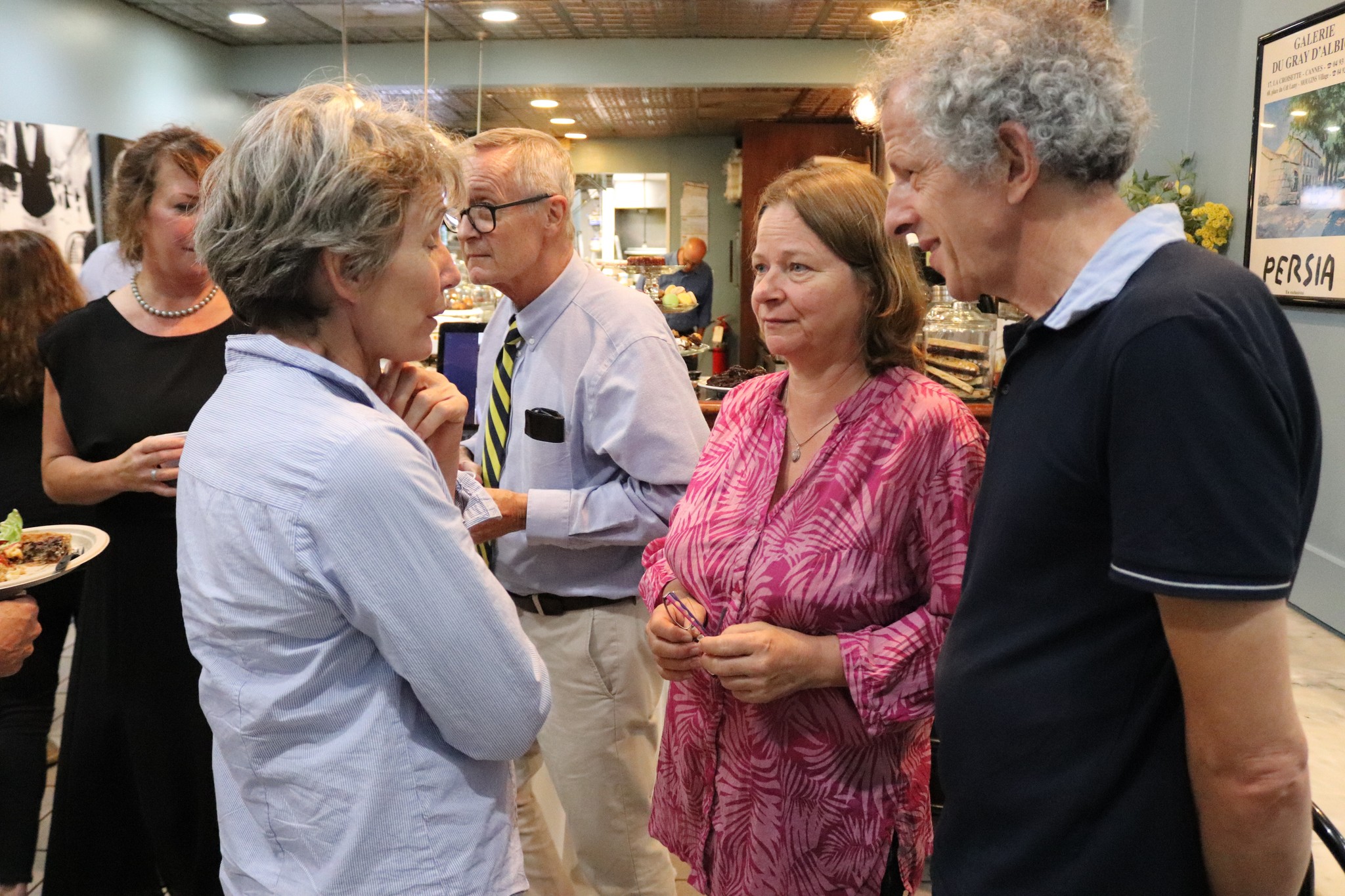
[[683, 251, 699, 267], [442, 193, 554, 233]]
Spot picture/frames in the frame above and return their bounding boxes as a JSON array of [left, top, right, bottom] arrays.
[[1241, 3, 1345, 309]]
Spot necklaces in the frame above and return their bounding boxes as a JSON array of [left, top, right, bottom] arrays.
[[782, 375, 872, 462], [130, 271, 220, 319]]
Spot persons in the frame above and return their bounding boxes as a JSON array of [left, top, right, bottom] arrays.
[[0, 124, 258, 896], [637, 237, 715, 372], [176, 79, 551, 896], [442, 128, 711, 896], [638, 157, 990, 896], [853, 0, 1324, 896]]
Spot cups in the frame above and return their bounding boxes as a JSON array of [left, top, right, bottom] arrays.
[[151, 431, 187, 488]]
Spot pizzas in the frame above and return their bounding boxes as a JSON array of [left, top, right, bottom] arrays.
[[0, 531, 71, 566]]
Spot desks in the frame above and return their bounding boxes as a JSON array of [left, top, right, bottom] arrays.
[[691, 383, 993, 430]]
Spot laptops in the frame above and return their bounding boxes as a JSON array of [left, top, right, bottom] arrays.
[[436, 323, 488, 441]]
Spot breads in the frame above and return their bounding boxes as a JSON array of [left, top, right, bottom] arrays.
[[447, 288, 474, 310]]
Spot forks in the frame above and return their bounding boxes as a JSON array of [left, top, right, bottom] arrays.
[[56, 547, 84, 571]]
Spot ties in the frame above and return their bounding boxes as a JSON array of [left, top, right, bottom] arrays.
[[475, 313, 523, 573]]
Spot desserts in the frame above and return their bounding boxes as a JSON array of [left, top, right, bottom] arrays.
[[627, 256, 665, 267], [658, 285, 697, 308], [672, 329, 703, 350], [706, 364, 767, 388]]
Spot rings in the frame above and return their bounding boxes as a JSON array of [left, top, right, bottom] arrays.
[[151, 468, 158, 482]]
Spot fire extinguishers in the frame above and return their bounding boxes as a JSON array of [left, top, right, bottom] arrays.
[[712, 314, 731, 376]]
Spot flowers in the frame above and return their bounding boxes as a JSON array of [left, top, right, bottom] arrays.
[[1130, 160, 1233, 255]]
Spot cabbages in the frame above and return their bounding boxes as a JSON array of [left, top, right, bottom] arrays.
[[0, 509, 23, 545]]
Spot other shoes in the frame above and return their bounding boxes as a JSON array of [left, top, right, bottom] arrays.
[[43, 738, 59, 766]]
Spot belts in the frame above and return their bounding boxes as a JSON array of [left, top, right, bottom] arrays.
[[506, 587, 634, 617]]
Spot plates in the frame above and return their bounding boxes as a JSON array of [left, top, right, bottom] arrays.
[[0, 525, 111, 593], [697, 380, 734, 393]]
